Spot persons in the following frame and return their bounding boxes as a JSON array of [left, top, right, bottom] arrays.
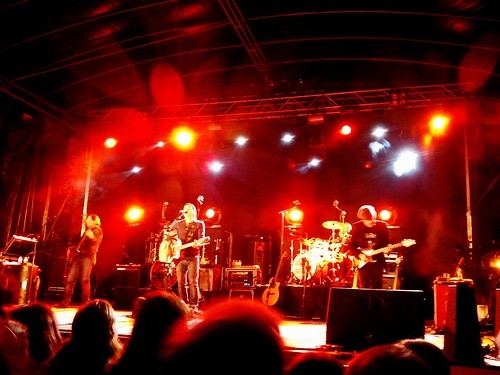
[[347, 205, 391, 289], [53, 214, 103, 308], [159, 202, 206, 314], [0, 289, 500, 375]]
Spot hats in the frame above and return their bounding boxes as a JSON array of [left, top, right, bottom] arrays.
[[357, 206, 376, 220]]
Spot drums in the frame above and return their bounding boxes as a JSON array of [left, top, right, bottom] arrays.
[[292, 238, 346, 281]]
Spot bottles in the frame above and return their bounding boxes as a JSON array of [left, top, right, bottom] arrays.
[[232, 259, 242, 268], [457, 267, 463, 280]]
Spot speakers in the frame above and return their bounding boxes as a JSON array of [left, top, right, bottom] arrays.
[[115, 269, 140, 288], [445, 283, 486, 368], [327, 287, 424, 350], [49, 257, 75, 289]]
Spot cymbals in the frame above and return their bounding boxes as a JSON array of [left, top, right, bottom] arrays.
[[323, 221, 344, 229], [285, 225, 301, 229]]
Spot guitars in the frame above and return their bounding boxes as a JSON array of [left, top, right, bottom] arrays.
[[159, 236, 210, 263], [262, 250, 289, 306], [64, 228, 92, 262], [352, 239, 416, 268]]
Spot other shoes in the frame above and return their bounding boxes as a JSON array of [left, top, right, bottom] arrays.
[[189, 305, 198, 310], [54, 302, 70, 308]]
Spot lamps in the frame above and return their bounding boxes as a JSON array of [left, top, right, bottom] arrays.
[[306, 115, 328, 125], [391, 91, 407, 108], [208, 122, 222, 130]]
